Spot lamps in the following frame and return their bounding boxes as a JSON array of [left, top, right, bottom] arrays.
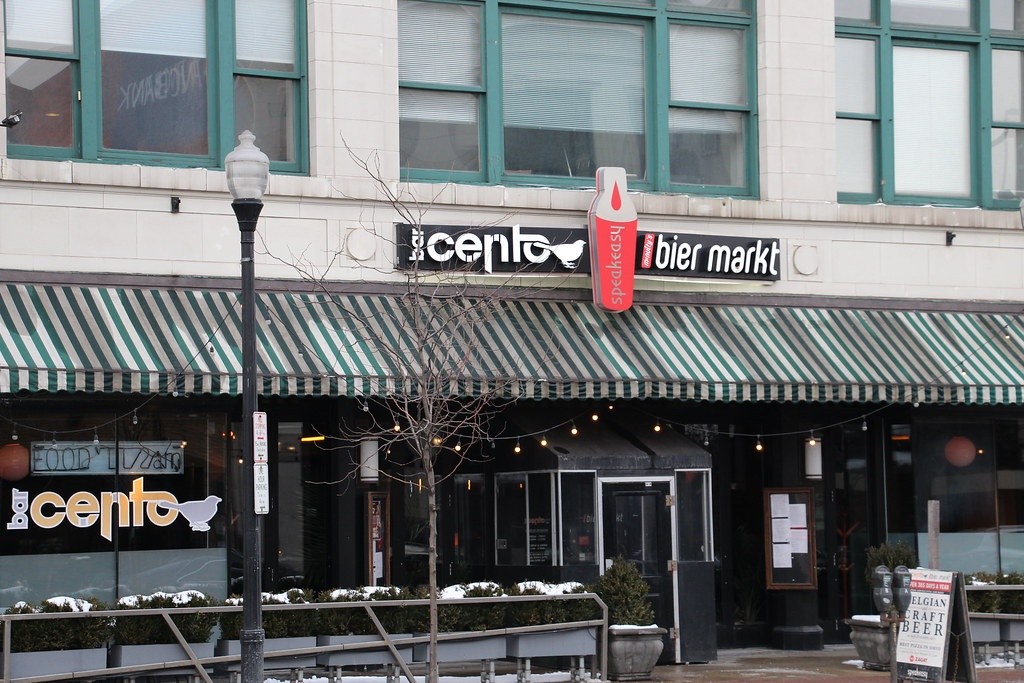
[[0, 442, 30, 481], [0, 110, 23, 129], [356, 434, 380, 488], [803, 431, 823, 482], [945, 435, 976, 468]]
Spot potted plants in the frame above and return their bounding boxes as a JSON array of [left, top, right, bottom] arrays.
[[0, 554, 667, 683], [845, 540, 1024, 672]]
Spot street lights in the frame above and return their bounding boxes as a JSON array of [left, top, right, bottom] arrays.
[[224, 130, 273, 683]]
